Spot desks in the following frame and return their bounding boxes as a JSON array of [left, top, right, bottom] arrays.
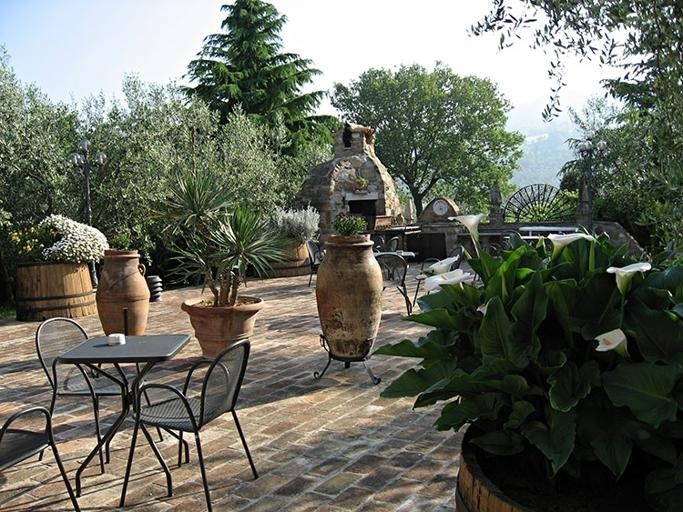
[[503, 226, 580, 246]]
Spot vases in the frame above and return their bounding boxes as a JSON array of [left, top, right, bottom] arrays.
[[97, 250, 151, 336], [455, 418, 544, 512], [315, 241, 384, 357]]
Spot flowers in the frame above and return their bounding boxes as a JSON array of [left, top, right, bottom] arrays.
[[8, 212, 130, 264], [275, 199, 321, 243], [366, 214, 683, 512], [327, 211, 371, 243]]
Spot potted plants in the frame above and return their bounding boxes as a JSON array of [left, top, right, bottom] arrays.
[[130, 161, 281, 361], [354, 176, 368, 189]]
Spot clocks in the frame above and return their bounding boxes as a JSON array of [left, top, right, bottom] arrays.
[[432, 200, 447, 216]]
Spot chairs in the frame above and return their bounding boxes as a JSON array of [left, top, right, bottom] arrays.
[[307, 230, 531, 318]]
